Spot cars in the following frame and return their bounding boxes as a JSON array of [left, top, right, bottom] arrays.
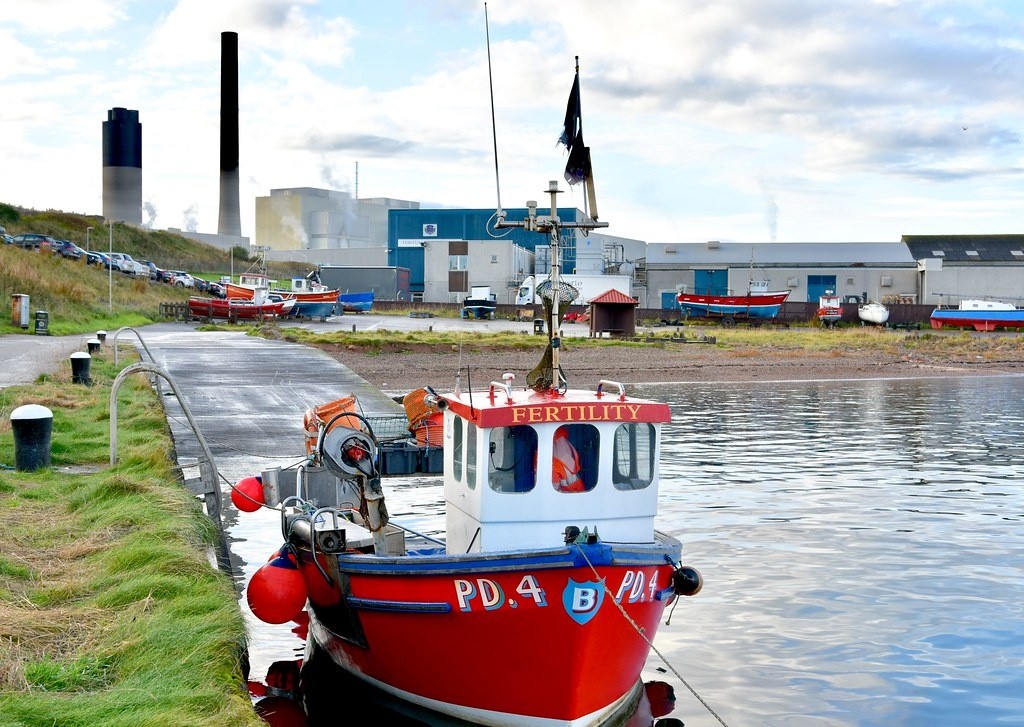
[[0, 226, 227, 298]]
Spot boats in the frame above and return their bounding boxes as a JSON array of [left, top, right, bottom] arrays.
[[231, 3, 703, 727], [462, 286, 497, 318], [817, 290, 844, 324], [676, 246, 792, 320], [930, 299, 1024, 331], [189, 246, 375, 319], [857, 300, 890, 323]]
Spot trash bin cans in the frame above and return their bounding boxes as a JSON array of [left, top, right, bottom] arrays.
[[34, 310, 49, 335]]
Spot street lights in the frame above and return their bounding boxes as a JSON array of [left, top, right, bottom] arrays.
[[87, 227, 94, 253], [109, 219, 125, 312]]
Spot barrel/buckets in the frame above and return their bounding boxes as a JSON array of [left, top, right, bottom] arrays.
[[315, 399, 360, 432], [403, 388, 443, 446], [12, 294, 30, 328]]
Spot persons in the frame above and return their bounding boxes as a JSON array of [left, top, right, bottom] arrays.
[[534, 426, 585, 493]]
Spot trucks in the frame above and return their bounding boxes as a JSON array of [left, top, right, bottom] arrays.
[[515, 274, 633, 305]]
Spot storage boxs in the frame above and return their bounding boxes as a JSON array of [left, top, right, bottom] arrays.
[[419, 446, 443, 473], [382, 447, 419, 475]]
